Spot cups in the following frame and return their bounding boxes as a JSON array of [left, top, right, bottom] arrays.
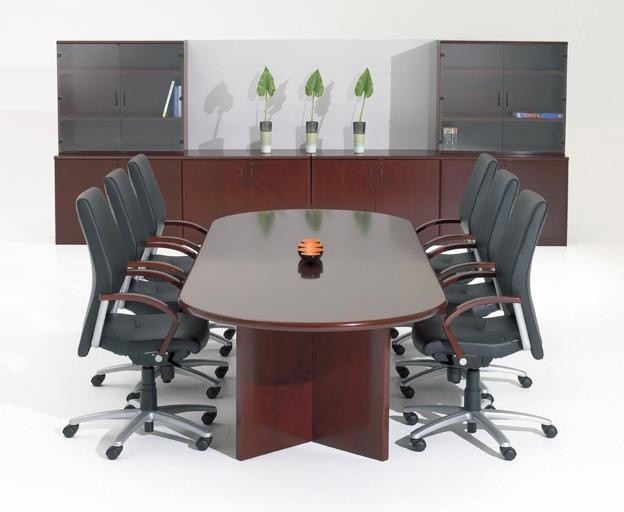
[[443, 128, 457, 150]]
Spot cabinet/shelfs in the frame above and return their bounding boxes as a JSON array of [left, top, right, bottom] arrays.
[[58, 41, 187, 155], [442, 158, 568, 247], [313, 159, 440, 245], [436, 40, 568, 155], [56, 160, 181, 243], [184, 160, 310, 252]]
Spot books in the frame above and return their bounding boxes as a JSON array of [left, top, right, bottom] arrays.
[[161, 80, 183, 119], [442, 127, 458, 150], [513, 109, 564, 120]]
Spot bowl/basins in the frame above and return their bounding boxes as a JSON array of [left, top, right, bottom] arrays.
[[298, 248, 323, 260], [300, 252, 320, 263], [301, 239, 319, 244], [298, 244, 323, 249]]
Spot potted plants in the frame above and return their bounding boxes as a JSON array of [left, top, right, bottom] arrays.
[[257, 66, 276, 154], [353, 68, 374, 154], [304, 68, 324, 154]]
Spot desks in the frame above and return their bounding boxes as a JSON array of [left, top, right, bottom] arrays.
[[179, 208, 449, 461]]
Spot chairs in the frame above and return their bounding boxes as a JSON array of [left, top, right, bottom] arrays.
[[390, 152, 500, 355], [403, 189, 558, 461], [396, 168, 533, 404], [91, 168, 230, 402], [63, 186, 218, 460], [127, 154, 235, 357]]
